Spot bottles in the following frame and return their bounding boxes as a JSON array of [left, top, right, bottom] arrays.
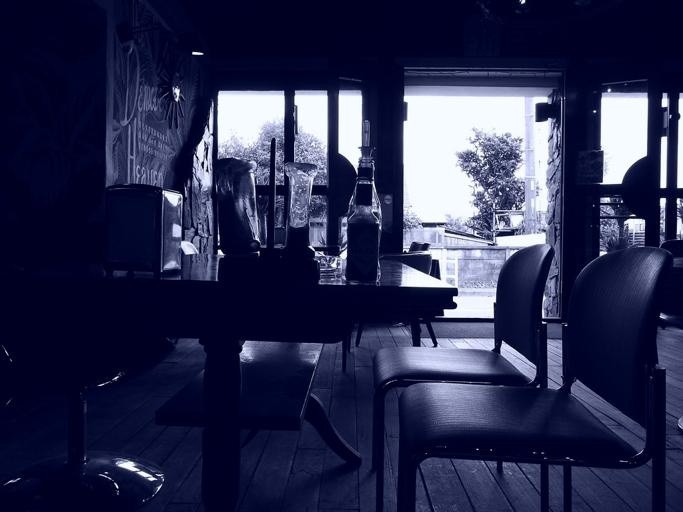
[[341, 145, 386, 287], [277, 159, 322, 280]]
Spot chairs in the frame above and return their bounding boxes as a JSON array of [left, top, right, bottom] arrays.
[[371, 243, 556, 512], [397, 246, 674, 512], [355, 249, 440, 348]]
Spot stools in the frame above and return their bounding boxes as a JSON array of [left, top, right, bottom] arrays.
[[0, 330, 166, 512]]
